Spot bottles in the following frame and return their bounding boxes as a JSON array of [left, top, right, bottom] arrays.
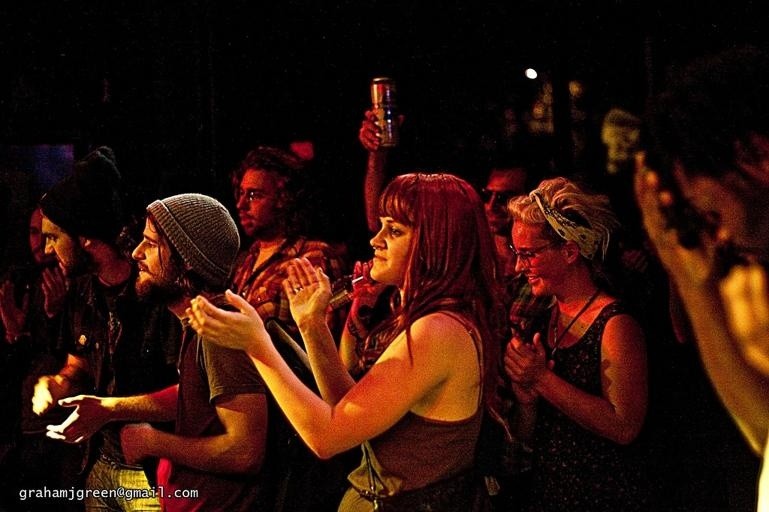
[[327, 274, 373, 311]]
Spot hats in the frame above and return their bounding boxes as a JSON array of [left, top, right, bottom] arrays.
[[146, 192, 241, 286], [39, 173, 125, 243]]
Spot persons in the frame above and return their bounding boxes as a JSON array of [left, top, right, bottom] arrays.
[[0, 109, 769, 512]]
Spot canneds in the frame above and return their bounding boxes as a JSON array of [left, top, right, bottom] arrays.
[[370, 77, 400, 151]]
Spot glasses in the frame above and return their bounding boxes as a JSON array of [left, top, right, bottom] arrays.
[[508, 240, 559, 260], [233, 187, 280, 204], [480, 188, 518, 204]]
